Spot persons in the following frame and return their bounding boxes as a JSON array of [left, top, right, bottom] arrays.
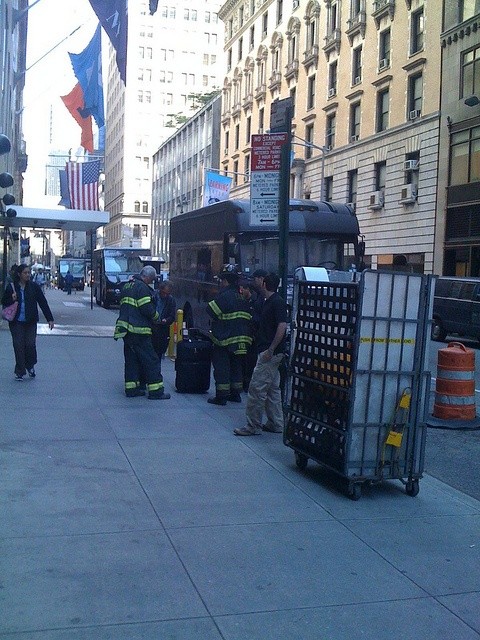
[[242, 278, 255, 321], [253, 269, 267, 337], [33, 268, 46, 295], [206, 272, 251, 405], [64, 270, 73, 295], [2, 264, 54, 381], [152, 279, 176, 361], [233, 272, 287, 435], [114, 265, 170, 399]]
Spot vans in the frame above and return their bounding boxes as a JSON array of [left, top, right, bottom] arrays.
[[431, 276, 480, 344]]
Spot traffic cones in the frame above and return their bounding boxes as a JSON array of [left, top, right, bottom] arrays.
[[433, 342, 475, 420]]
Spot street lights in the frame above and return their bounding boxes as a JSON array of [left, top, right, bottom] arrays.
[[266, 129, 325, 202]]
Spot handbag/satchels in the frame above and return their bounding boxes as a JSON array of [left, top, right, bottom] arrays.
[[0, 282, 18, 322]]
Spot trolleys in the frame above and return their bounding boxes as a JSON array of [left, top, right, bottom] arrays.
[[283, 266, 438, 500]]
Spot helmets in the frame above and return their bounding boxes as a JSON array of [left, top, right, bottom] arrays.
[[218, 264, 238, 278]]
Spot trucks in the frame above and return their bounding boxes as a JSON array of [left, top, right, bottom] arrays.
[[94, 249, 151, 309], [170, 199, 366, 386], [58, 260, 85, 291]]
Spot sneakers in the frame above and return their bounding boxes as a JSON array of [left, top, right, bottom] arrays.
[[126, 388, 145, 397], [28, 367, 35, 377], [207, 397, 227, 405], [228, 395, 242, 402], [236, 426, 262, 436], [260, 422, 277, 432], [16, 375, 23, 381], [148, 393, 171, 399]]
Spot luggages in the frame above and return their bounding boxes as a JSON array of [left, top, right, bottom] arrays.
[[175, 328, 212, 393]]
